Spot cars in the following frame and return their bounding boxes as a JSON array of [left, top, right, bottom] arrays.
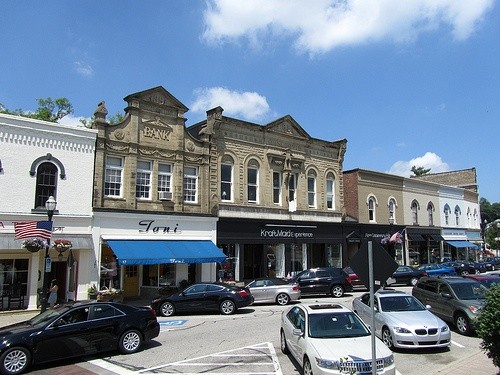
[[431, 247, 440, 258], [279, 300, 396, 375], [243, 276, 302, 306], [342, 265, 365, 285], [416, 249, 500, 289], [150, 281, 255, 317], [352, 288, 452, 352], [0, 299, 160, 375], [409, 248, 421, 259], [391, 265, 430, 287], [287, 267, 354, 298]]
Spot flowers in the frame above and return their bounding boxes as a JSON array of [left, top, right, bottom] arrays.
[[52, 240, 72, 248], [22, 238, 49, 248]]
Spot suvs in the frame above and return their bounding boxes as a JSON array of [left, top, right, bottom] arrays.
[[411, 275, 490, 336]]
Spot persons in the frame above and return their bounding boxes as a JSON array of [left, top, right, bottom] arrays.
[[61, 310, 83, 324], [47, 279, 59, 308]]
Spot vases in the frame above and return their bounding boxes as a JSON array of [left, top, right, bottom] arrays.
[[55, 247, 68, 252], [25, 246, 40, 252]]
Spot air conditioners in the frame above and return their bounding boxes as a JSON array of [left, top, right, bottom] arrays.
[[158, 192, 173, 200]]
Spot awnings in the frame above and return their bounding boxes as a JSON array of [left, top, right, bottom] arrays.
[[446, 241, 478, 249], [108, 241, 227, 264]]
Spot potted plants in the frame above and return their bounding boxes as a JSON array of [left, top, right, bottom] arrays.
[[88, 284, 98, 299]]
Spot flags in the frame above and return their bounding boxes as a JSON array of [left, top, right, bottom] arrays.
[[14, 220, 52, 240]]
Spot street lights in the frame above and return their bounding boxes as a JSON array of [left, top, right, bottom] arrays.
[[40, 194, 58, 314]]
